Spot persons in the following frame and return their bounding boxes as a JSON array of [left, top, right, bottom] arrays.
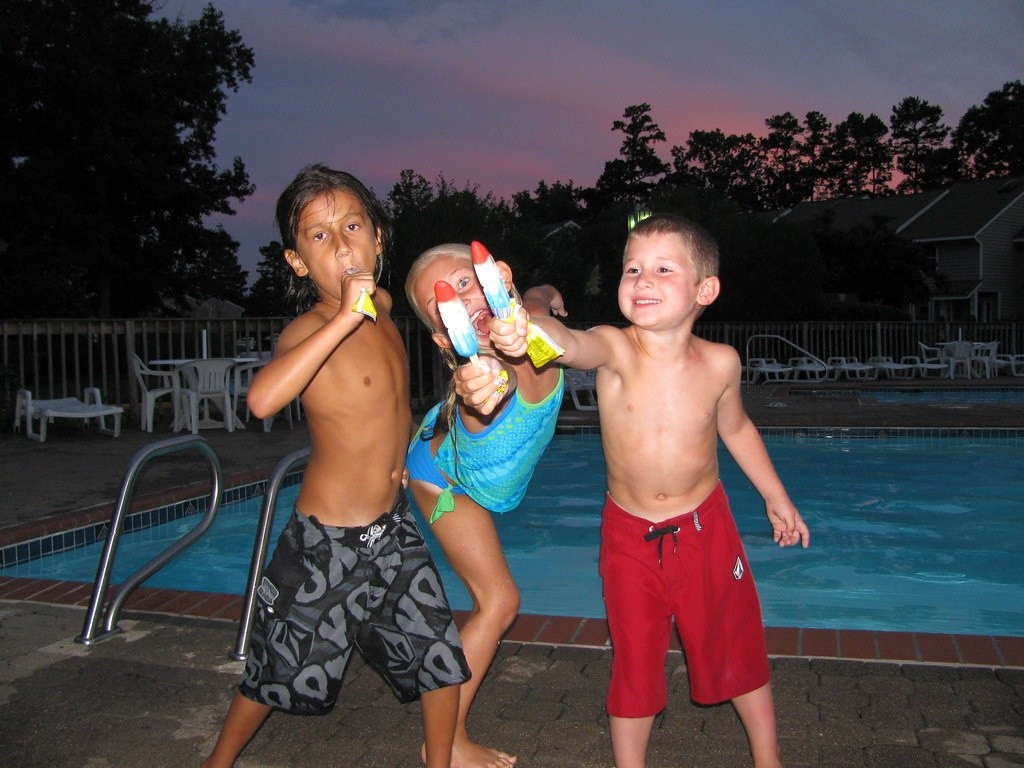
[[487, 214, 810, 768], [203, 159, 473, 768], [405, 244, 568, 768]]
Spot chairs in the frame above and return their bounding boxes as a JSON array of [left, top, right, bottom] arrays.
[[129, 352, 302, 433], [14, 385, 124, 443], [740, 339, 1024, 384]]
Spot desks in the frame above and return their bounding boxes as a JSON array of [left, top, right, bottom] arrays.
[[148, 358, 259, 433], [935, 342, 985, 379]]
